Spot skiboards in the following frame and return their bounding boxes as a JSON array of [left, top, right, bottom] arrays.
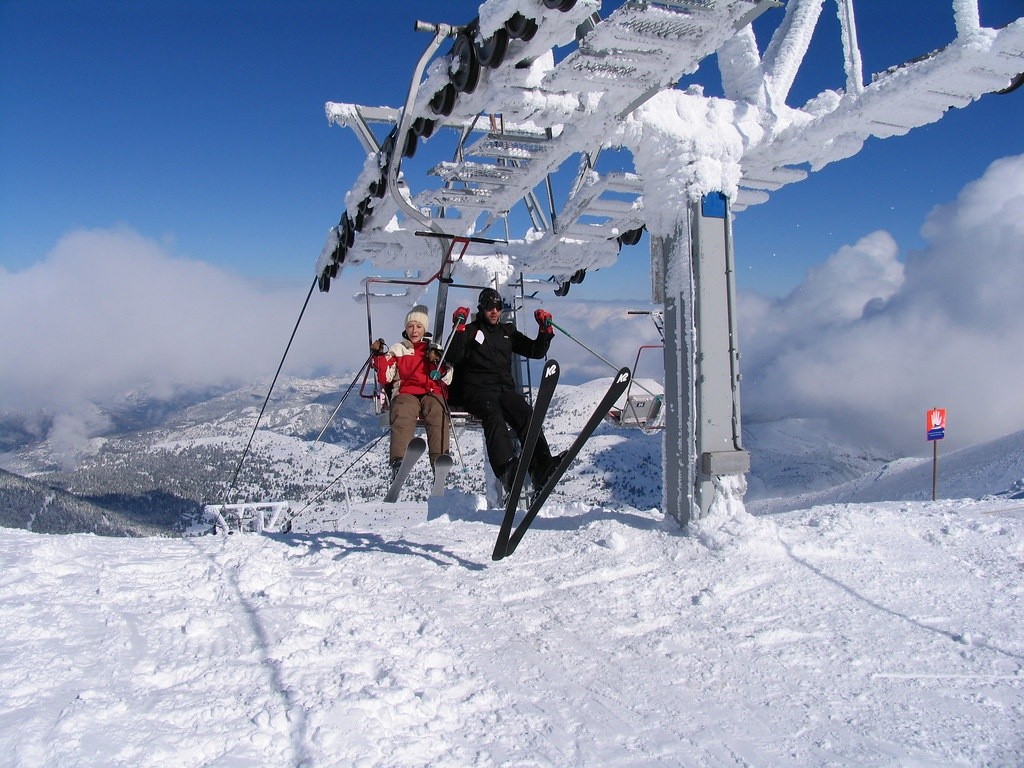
[[382, 436, 454, 504], [490, 358, 632, 561]]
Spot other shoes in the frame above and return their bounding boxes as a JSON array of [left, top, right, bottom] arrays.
[[500, 457, 519, 494]]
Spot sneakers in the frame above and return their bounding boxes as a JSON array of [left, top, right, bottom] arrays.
[[392, 458, 403, 480]]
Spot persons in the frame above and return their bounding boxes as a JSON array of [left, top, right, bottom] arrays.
[[371, 304, 453, 486], [444, 287, 569, 497]]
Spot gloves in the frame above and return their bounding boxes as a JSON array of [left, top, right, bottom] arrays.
[[452, 307, 470, 333], [371, 340, 384, 355], [429, 349, 446, 365], [534, 309, 553, 334]]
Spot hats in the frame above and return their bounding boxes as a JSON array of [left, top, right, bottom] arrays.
[[405, 305, 429, 333], [477, 288, 504, 311]]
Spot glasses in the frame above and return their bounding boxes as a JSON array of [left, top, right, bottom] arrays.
[[483, 301, 504, 312]]
